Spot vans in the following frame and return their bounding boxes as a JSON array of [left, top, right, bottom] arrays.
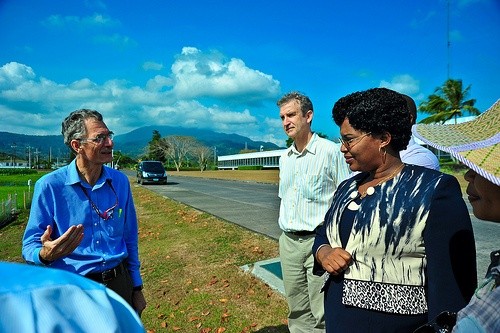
[[137, 160, 168, 185]]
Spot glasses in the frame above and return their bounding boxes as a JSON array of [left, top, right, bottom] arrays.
[[338, 132, 371, 150], [76, 132, 115, 143], [413, 310, 457, 333]]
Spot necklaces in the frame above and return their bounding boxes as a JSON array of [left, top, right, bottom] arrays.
[[360, 164, 404, 200]]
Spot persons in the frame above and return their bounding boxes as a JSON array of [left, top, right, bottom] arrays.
[[311, 87, 479, 333], [0, 259, 147, 333], [399, 94, 440, 171], [21, 109, 147, 317], [277, 91, 352, 333], [411, 99, 500, 333]]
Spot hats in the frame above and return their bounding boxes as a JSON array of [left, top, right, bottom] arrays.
[[411, 98, 500, 187]]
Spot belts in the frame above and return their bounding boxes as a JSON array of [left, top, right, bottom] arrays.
[[85, 261, 126, 282], [286, 227, 321, 236]]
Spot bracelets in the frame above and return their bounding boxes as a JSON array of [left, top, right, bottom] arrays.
[[315, 244, 331, 263]]
